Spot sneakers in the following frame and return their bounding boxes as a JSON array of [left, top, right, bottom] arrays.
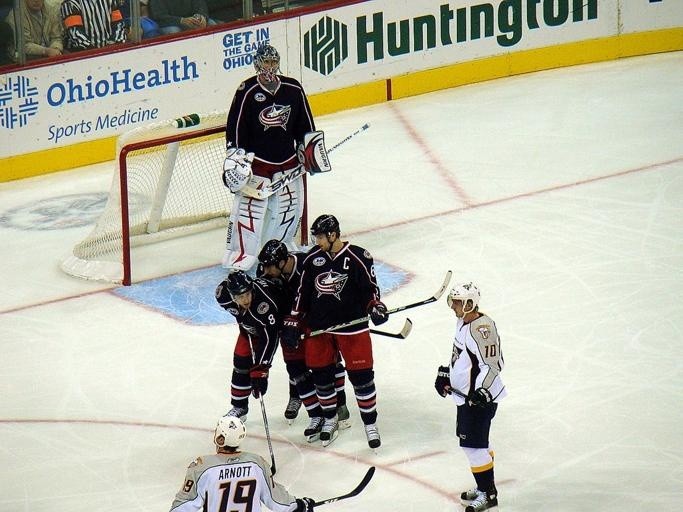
[[319, 416, 340, 441], [461, 487, 479, 501], [465, 493, 499, 512], [284, 398, 303, 420], [223, 407, 248, 418], [336, 407, 351, 421], [303, 417, 326, 436], [364, 423, 381, 448]]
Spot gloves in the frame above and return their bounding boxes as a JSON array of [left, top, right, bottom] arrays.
[[297, 130, 331, 175], [366, 300, 389, 326], [466, 388, 494, 411], [280, 317, 311, 352], [434, 365, 453, 399], [220, 146, 256, 194], [247, 366, 273, 399], [293, 497, 318, 512]]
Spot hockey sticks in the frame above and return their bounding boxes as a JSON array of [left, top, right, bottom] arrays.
[[247, 332, 276, 477], [239, 122, 371, 201], [368, 318, 412, 340], [449, 385, 506, 411], [311, 466, 376, 509], [300, 269, 453, 339]]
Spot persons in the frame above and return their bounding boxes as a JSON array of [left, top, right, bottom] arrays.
[[147, 0, 216, 34], [281, 213, 389, 448], [169, 415, 315, 512], [215, 271, 322, 444], [60, 0, 128, 51], [222, 45, 332, 271], [5, 0, 71, 60], [435, 282, 505, 511], [254, 240, 351, 429]]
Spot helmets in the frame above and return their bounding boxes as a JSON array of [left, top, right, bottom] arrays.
[[311, 212, 342, 234], [253, 44, 282, 84], [211, 416, 247, 449], [258, 239, 289, 267], [224, 269, 254, 295], [446, 281, 483, 306]]
